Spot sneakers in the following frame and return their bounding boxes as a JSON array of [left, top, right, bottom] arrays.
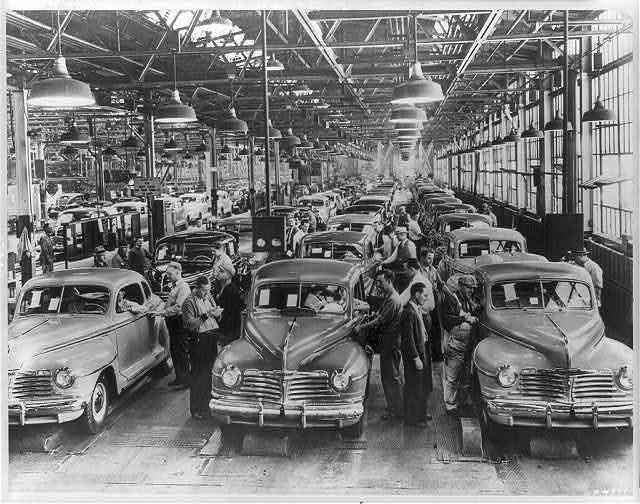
[[168, 375, 190, 390], [191, 409, 210, 421], [380, 401, 472, 429]]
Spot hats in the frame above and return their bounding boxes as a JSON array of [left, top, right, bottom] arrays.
[[393, 225, 409, 234], [567, 243, 591, 256], [92, 244, 109, 256], [458, 274, 479, 288]]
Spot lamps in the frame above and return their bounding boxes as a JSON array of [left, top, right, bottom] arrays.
[[390, 101, 428, 163], [546, 41, 574, 131], [254, 91, 378, 163], [582, 12, 616, 122], [31, 113, 265, 161], [28, 9, 96, 107], [154, 50, 198, 124], [391, 16, 445, 105], [220, 75, 249, 134], [522, 75, 544, 138], [435, 106, 522, 160]]
